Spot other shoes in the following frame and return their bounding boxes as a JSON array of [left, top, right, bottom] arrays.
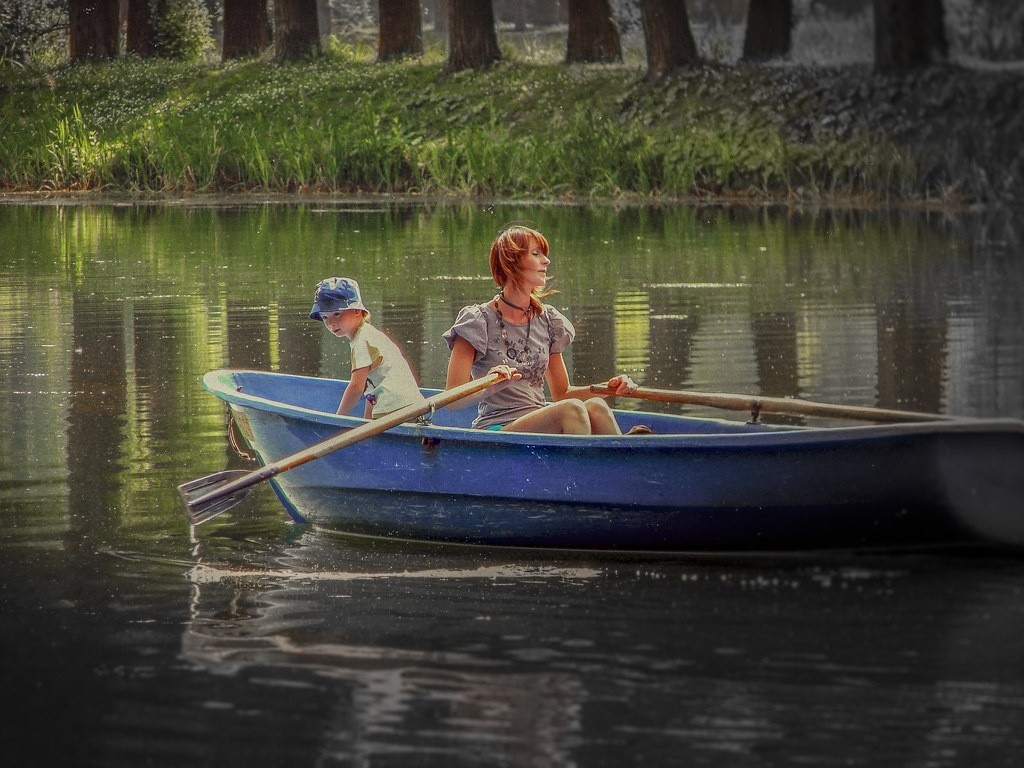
[[625, 425, 652, 434]]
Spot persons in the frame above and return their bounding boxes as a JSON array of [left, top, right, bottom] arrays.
[[307, 275, 426, 427], [443, 224, 652, 436]]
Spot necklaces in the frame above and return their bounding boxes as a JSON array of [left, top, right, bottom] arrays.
[[493, 291, 534, 362]]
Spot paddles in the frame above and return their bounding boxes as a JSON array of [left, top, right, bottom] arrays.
[[587, 382, 961, 424], [174, 366, 520, 527]]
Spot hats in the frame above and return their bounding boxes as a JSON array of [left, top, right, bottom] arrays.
[[309, 277, 369, 321]]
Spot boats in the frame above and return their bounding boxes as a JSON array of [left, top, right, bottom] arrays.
[[200, 368, 1023, 568]]
